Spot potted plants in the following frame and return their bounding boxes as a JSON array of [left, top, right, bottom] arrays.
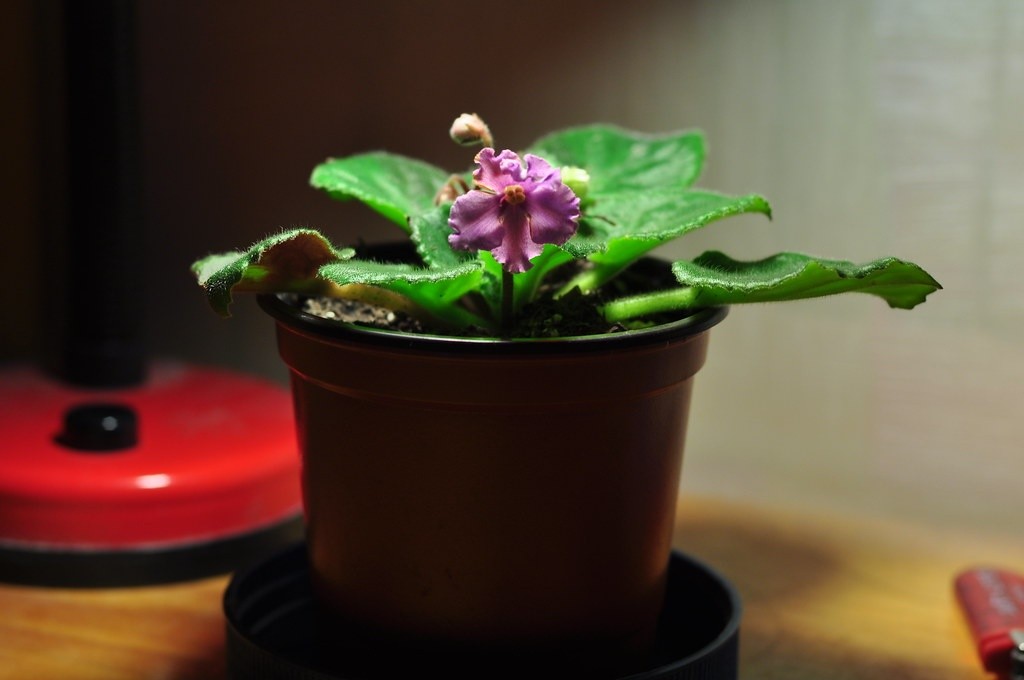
[[256, 239, 727, 680]]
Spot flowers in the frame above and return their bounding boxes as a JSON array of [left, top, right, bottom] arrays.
[[189, 113, 943, 337]]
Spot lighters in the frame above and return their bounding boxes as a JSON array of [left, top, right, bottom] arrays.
[[954, 564, 1024, 680]]
